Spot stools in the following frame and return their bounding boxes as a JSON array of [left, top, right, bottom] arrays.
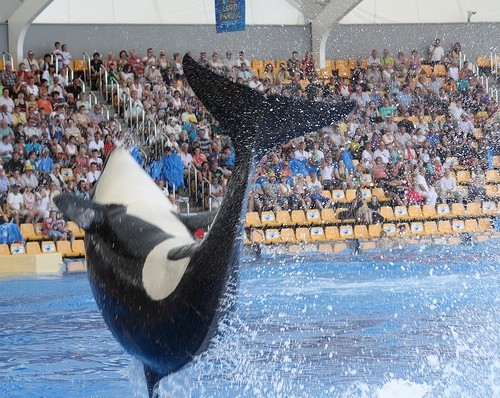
[[1, 57, 499, 276]]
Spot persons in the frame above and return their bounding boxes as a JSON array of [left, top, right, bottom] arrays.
[[0, 38, 500, 248]]
